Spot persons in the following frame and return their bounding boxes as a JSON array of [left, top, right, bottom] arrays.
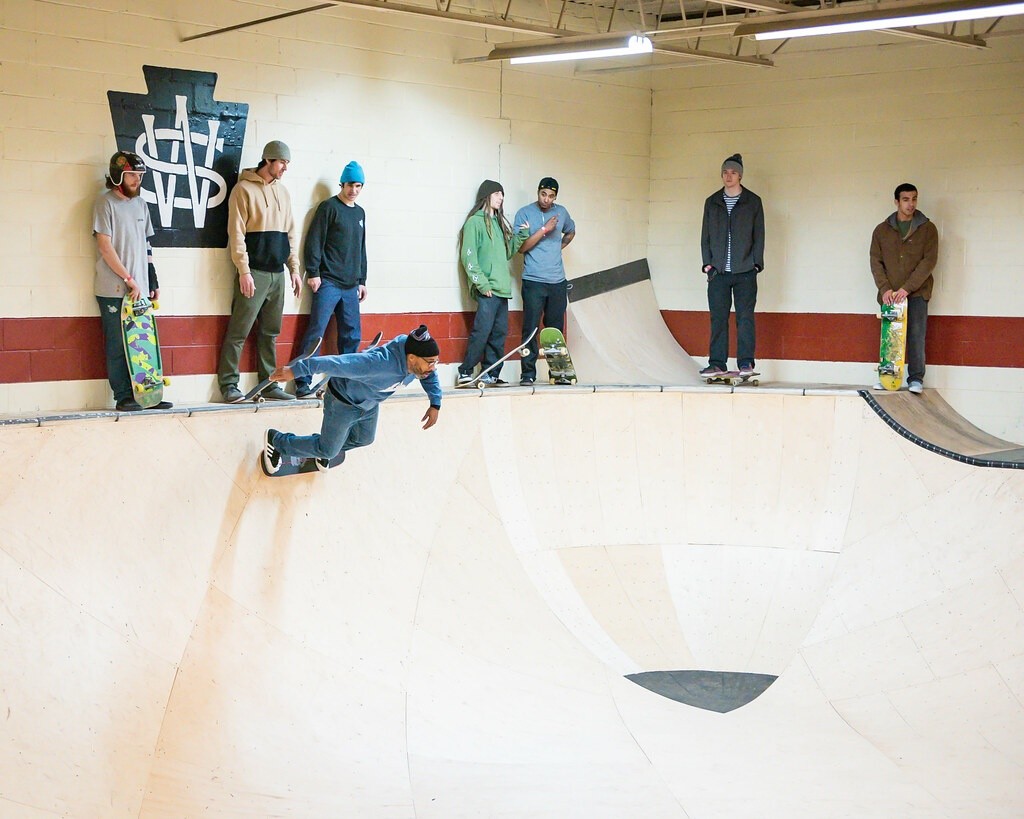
[[698, 155, 766, 376], [295, 160, 371, 395], [512, 175, 575, 386], [456, 179, 532, 390], [264, 320, 443, 480], [215, 139, 298, 407], [868, 182, 940, 393], [89, 150, 174, 412]]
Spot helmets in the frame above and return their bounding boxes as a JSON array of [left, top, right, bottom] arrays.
[[107, 151, 147, 186]]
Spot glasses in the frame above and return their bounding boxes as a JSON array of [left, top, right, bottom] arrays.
[[419, 356, 440, 366]]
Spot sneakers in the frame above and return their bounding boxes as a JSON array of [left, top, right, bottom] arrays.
[[738, 361, 753, 376], [312, 433, 330, 473], [519, 376, 534, 385], [699, 365, 728, 377], [456, 373, 472, 385], [478, 376, 510, 387], [145, 401, 174, 409], [116, 398, 143, 411], [295, 383, 311, 397], [221, 383, 246, 403], [260, 388, 297, 401], [908, 381, 923, 394], [873, 383, 886, 390], [264, 427, 282, 475]]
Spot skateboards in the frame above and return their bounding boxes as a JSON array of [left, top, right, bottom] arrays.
[[227, 336, 322, 403], [539, 327, 577, 385], [264, 450, 345, 477], [299, 330, 383, 397], [875, 292, 907, 390], [122, 291, 171, 407], [701, 371, 760, 387], [455, 325, 538, 389]]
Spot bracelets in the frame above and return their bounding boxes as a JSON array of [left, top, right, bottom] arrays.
[[124, 275, 132, 283], [541, 226, 550, 234]]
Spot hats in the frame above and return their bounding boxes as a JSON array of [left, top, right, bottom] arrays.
[[404, 324, 440, 358], [721, 153, 743, 176], [475, 179, 505, 202], [340, 162, 365, 185], [262, 141, 291, 162], [539, 177, 559, 193]]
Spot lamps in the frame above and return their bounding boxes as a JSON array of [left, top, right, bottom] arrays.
[[729, 0, 1024, 47], [474, 0, 655, 64]]
